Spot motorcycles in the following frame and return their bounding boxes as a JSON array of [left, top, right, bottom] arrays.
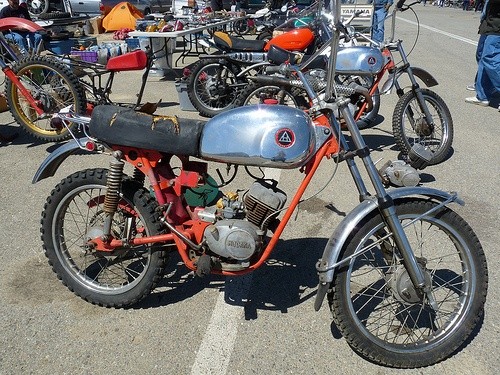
[[32, 0, 491, 369], [0, 16, 87, 143], [231, 0, 456, 164], [186, 0, 382, 131]]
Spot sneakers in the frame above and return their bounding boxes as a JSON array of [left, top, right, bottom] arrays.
[[467, 83, 476, 91], [465, 96, 489, 107]]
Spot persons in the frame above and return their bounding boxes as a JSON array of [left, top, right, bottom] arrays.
[[462, 0, 500, 109], [366, 0, 393, 46], [424, 0, 461, 11], [0, 0, 37, 52]]
